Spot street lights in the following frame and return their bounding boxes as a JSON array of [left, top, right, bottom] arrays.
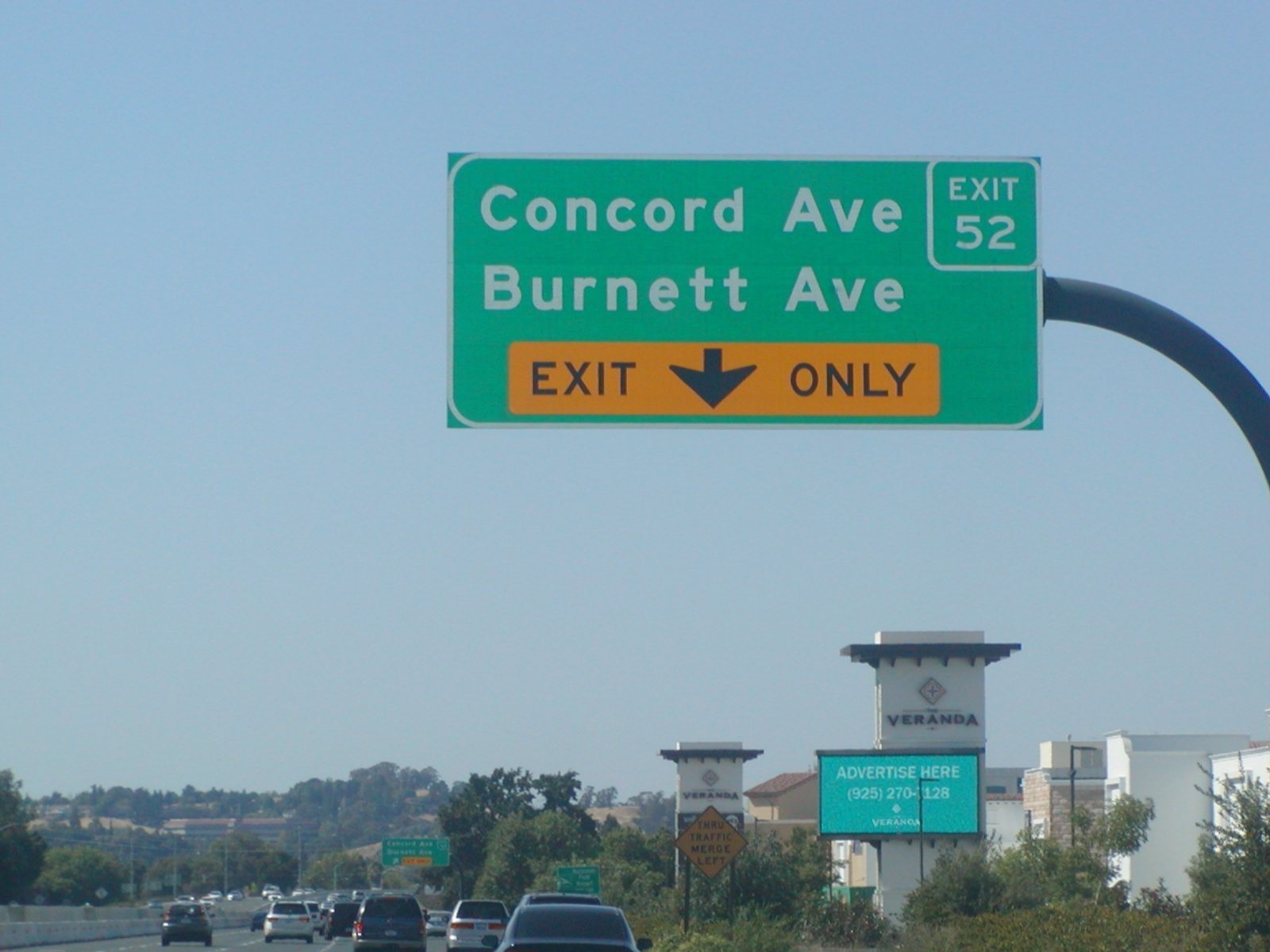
[[917, 777, 942, 890], [754, 816, 770, 849], [1067, 741, 1101, 897]]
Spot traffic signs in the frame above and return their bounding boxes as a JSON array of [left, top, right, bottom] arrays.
[[381, 838, 451, 868], [445, 150, 1046, 432]]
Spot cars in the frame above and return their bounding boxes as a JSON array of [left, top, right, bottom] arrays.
[[441, 898, 511, 952], [158, 901, 216, 946], [480, 902, 655, 952], [148, 883, 374, 941], [511, 891, 602, 909], [424, 910, 452, 937], [262, 899, 318, 944]]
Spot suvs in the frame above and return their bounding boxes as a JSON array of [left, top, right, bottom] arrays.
[[351, 893, 431, 952]]
